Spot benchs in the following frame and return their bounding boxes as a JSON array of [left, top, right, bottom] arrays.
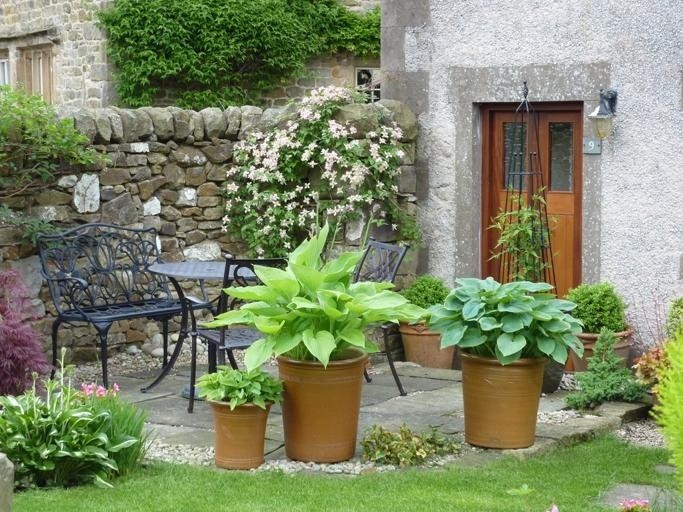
[[34, 224, 214, 396]]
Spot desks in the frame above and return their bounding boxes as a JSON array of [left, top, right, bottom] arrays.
[[143, 256, 259, 399]]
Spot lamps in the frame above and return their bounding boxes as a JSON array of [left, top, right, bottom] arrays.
[[590, 88, 618, 139]]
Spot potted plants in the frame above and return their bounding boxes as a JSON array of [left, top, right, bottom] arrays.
[[193, 365, 286, 472], [425, 276, 586, 450], [397, 275, 455, 370], [192, 218, 431, 463], [562, 282, 634, 372]]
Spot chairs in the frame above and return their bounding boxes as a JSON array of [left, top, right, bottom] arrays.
[[185, 258, 289, 413]]
